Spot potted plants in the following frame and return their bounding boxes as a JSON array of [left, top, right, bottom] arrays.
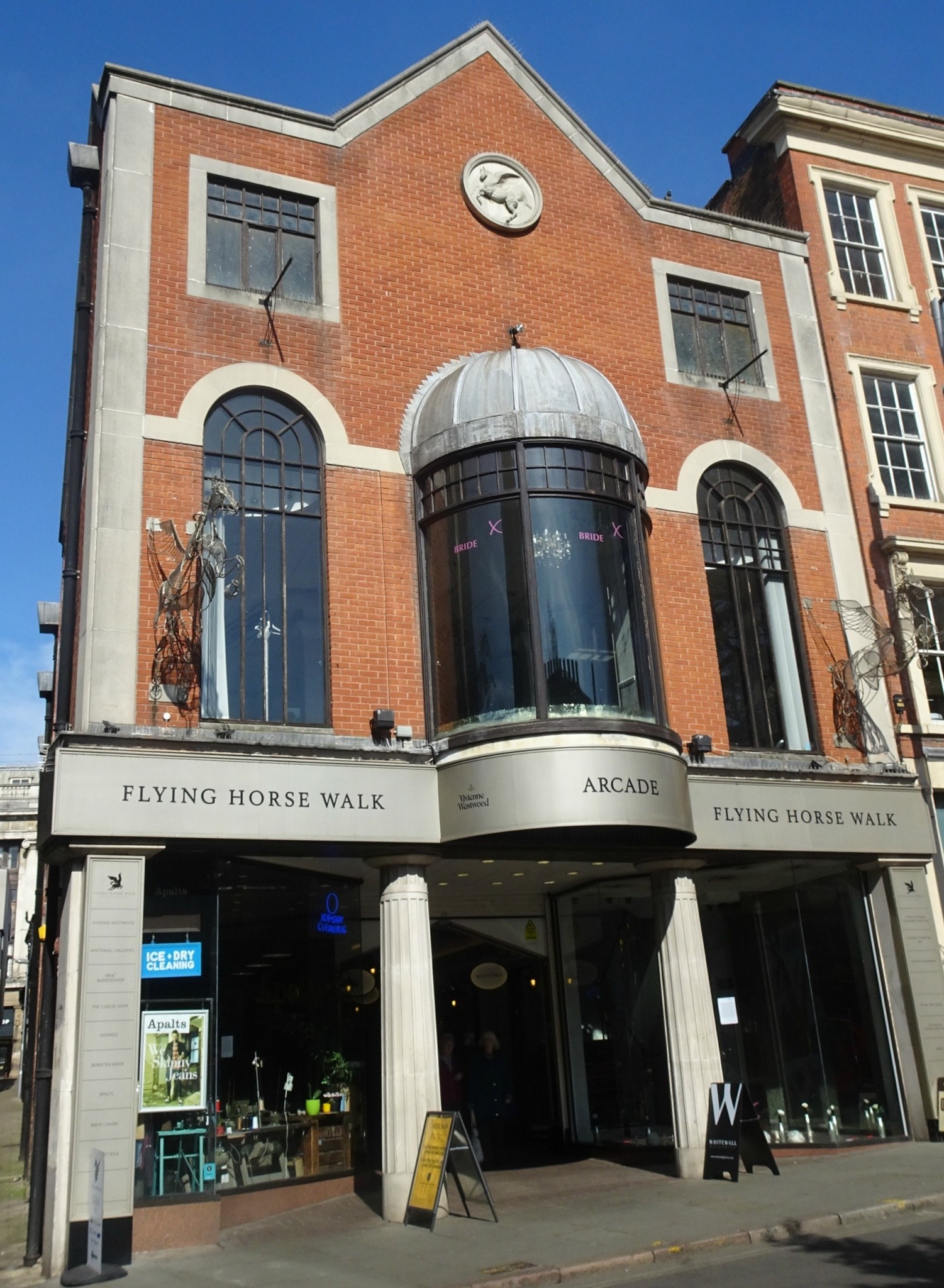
[[306, 1084, 322, 1114], [323, 1053, 350, 1111]]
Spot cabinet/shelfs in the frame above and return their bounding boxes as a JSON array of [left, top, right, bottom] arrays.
[[157, 1127, 208, 1195]]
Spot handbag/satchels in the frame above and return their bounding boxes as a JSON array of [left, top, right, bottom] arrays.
[[460, 1109, 484, 1167]]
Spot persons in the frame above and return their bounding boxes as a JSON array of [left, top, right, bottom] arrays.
[[469, 1031, 511, 1169], [163, 1031, 189, 1104], [439, 1033, 469, 1172]]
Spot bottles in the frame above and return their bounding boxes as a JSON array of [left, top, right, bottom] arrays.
[[217, 1096, 220, 1111], [259, 1096, 264, 1111]]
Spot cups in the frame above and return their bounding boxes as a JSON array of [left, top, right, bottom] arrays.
[[252, 1116, 258, 1129]]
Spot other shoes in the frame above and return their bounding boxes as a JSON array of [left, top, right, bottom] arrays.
[[177, 1098, 182, 1103], [164, 1097, 170, 1103]]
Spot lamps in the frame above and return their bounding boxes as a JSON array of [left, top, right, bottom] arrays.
[[691, 733, 711, 755], [370, 709, 395, 730]]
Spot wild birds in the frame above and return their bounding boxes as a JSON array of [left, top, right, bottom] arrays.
[[665, 190, 672, 201]]
